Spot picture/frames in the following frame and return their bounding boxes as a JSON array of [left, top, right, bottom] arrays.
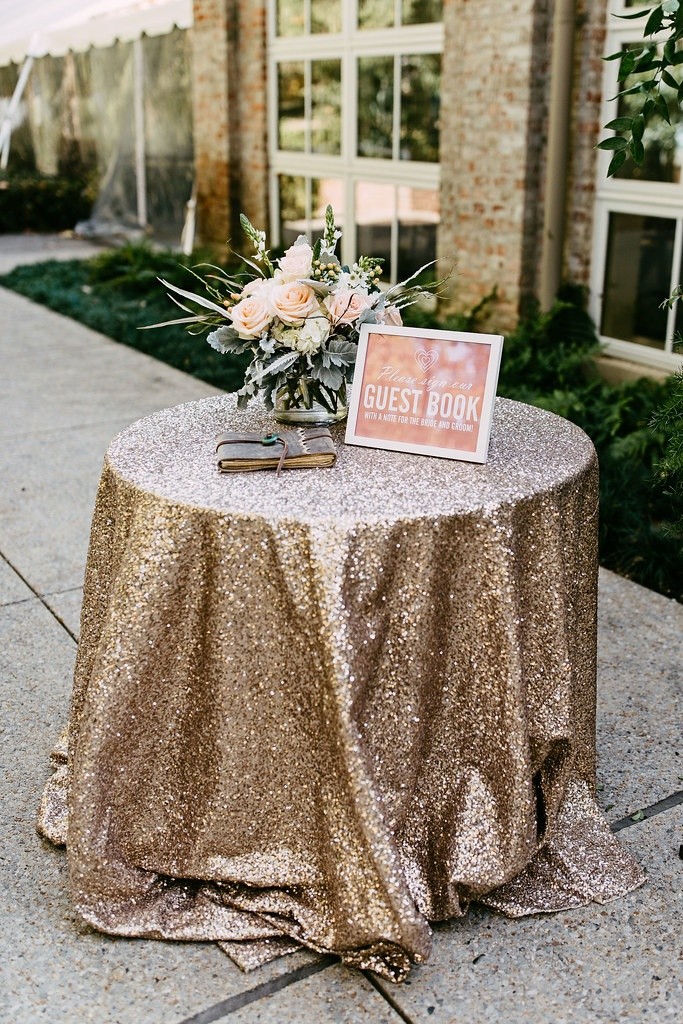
[[344, 322, 505, 463]]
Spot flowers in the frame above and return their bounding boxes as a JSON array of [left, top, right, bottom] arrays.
[[135, 204, 462, 415]]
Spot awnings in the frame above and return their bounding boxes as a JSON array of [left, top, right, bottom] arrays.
[[0, 0, 196, 69]]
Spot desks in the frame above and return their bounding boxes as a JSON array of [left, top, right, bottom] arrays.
[[105, 390, 600, 526]]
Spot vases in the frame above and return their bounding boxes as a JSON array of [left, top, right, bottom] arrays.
[[272, 385, 349, 428]]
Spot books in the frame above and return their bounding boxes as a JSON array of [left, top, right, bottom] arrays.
[[214, 426, 338, 474]]
